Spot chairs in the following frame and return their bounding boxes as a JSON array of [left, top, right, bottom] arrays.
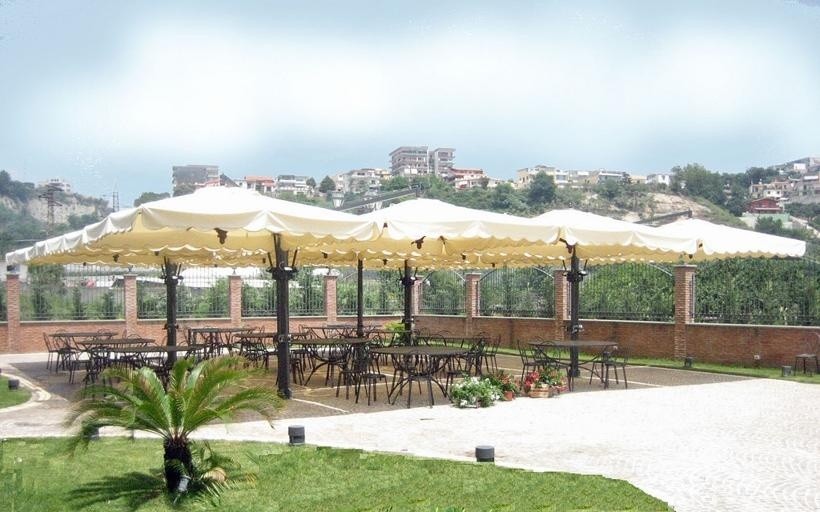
[[37, 322, 628, 408]]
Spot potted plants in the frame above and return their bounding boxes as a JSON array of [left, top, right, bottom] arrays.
[[61, 353, 284, 498]]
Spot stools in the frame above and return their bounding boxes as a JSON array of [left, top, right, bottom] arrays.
[[795, 354, 819, 377]]
